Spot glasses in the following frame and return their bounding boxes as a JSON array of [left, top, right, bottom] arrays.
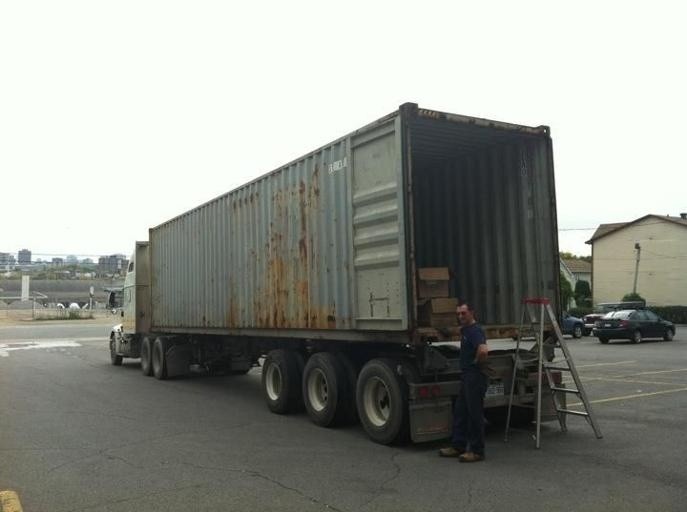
[[457, 311, 466, 316]]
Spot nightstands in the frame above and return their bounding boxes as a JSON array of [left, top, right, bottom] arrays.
[[412, 265, 460, 329]]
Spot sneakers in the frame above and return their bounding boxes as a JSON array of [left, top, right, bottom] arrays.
[[440, 447, 485, 462]]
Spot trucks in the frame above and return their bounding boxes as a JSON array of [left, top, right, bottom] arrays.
[[108, 102, 567, 445]]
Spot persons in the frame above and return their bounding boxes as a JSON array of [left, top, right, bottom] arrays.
[[439, 301, 496, 461]]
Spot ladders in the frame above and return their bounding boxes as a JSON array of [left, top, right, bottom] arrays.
[[502, 298, 603, 449]]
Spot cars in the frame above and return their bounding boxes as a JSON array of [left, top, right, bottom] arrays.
[[562, 306, 676, 344]]
